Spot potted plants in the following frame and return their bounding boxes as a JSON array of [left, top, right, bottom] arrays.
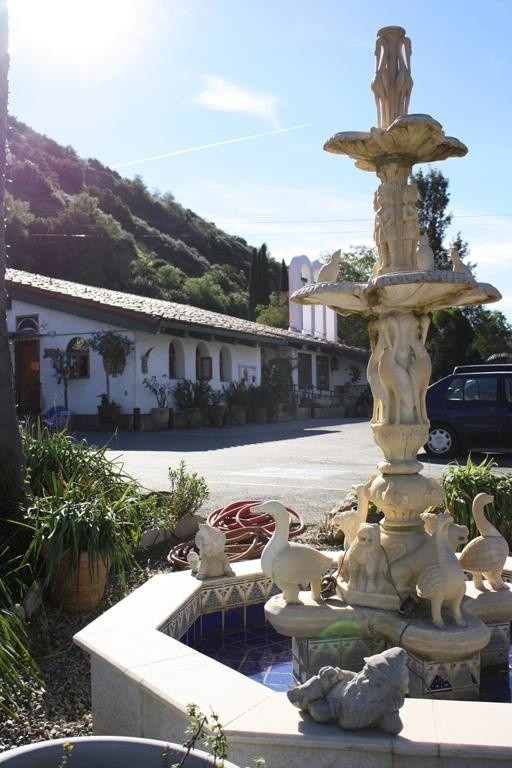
[[2, 391, 173, 616], [41, 325, 137, 436], [142, 364, 286, 431], [0, 699, 267, 768]]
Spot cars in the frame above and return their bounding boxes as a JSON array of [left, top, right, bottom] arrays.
[[422, 362, 511, 459]]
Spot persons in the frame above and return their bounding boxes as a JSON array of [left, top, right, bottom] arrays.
[[373, 183, 420, 276], [285, 646, 409, 735]]
